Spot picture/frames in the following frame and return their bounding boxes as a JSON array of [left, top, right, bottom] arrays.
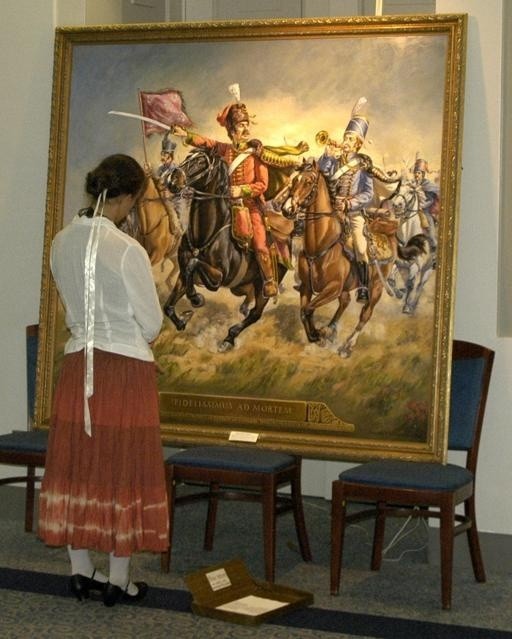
[[32, 10, 470, 467]]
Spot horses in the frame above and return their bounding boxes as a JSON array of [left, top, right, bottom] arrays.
[[117, 140, 438, 360]]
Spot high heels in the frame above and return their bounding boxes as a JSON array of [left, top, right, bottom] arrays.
[[104, 580, 147, 607], [71, 567, 109, 600]]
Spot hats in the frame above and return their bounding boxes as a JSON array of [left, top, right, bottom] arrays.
[[216, 104, 249, 128], [344, 115, 369, 140], [414, 159, 427, 172], [161, 139, 176, 153]]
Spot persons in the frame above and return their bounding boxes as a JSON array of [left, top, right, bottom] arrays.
[[37, 154, 172, 605]]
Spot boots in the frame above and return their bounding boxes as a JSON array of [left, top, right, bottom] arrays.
[[356, 261, 368, 303], [256, 252, 276, 296]]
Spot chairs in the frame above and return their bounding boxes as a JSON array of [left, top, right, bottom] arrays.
[[330, 337, 495, 612], [2, 321, 52, 529], [160, 442, 315, 583]]
